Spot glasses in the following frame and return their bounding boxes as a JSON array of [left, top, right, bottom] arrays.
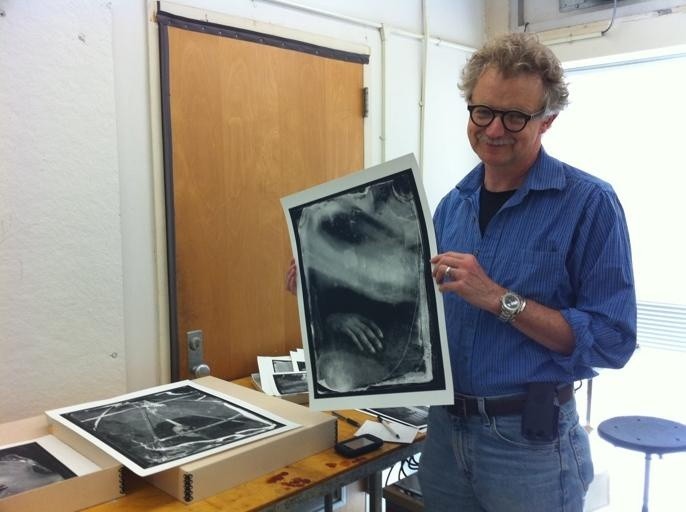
[[468, 99, 545, 133]]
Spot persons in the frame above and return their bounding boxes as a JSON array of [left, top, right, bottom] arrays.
[[327, 312, 384, 353], [286, 34, 637, 512]]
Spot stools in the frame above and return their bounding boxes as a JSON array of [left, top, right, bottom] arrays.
[[599, 414, 686, 510]]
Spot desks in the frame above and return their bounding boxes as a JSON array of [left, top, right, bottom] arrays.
[[1, 374, 429, 512]]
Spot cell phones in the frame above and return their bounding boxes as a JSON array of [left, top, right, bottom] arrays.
[[335, 434, 383, 458]]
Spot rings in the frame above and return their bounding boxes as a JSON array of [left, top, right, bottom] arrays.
[[446, 266, 451, 277]]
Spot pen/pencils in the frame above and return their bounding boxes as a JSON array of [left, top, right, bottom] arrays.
[[332, 411, 360, 428], [377, 415, 400, 440]]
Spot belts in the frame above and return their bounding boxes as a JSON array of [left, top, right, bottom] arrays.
[[448, 383, 573, 418]]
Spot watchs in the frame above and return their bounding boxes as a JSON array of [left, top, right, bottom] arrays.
[[498, 290, 526, 324]]
